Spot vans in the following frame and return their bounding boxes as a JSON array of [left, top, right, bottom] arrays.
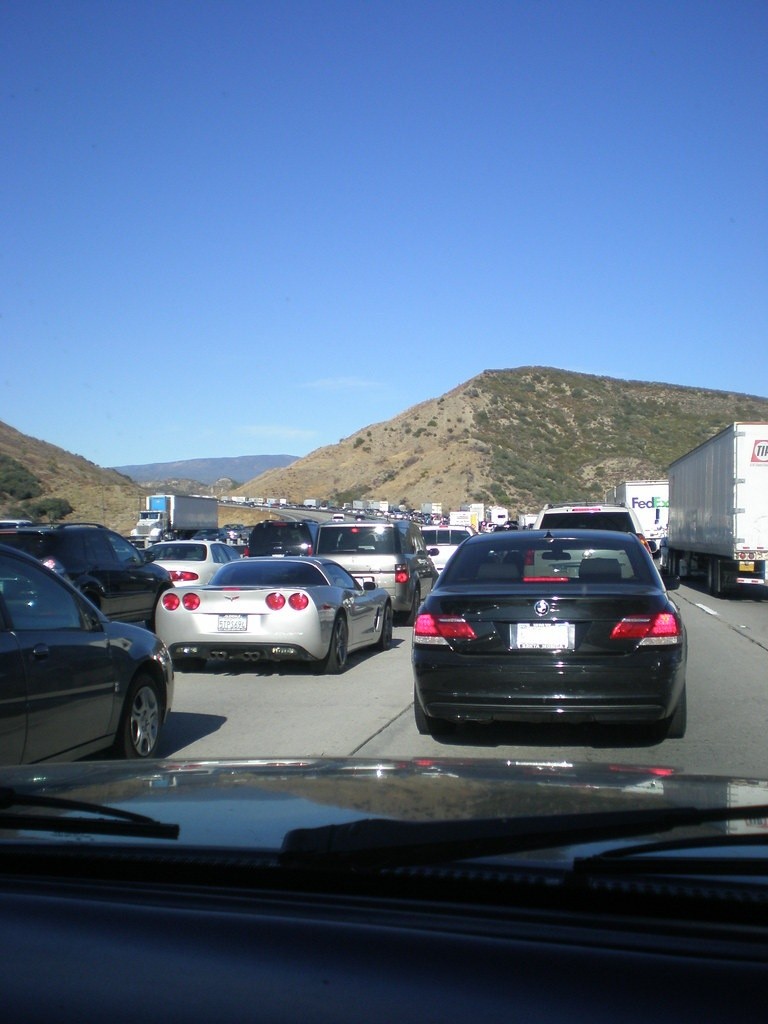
[[314, 521, 439, 626]]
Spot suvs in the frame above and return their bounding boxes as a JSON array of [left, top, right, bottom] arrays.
[[524, 502, 654, 578], [0, 522, 175, 633], [244, 519, 318, 556]]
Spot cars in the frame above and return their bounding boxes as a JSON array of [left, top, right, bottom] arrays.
[[411, 527, 688, 740], [190, 529, 226, 543], [0, 539, 174, 762], [242, 526, 255, 540], [222, 523, 243, 541], [140, 537, 243, 590]]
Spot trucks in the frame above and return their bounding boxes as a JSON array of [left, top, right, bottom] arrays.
[[606, 480, 670, 559], [131, 495, 219, 545], [221, 495, 537, 576]]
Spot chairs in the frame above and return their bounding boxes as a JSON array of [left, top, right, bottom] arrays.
[[580, 558, 623, 579]]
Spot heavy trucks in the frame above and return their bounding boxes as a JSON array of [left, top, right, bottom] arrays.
[[661, 421, 768, 596]]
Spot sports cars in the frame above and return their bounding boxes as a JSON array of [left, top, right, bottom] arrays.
[[156, 555, 395, 674]]
